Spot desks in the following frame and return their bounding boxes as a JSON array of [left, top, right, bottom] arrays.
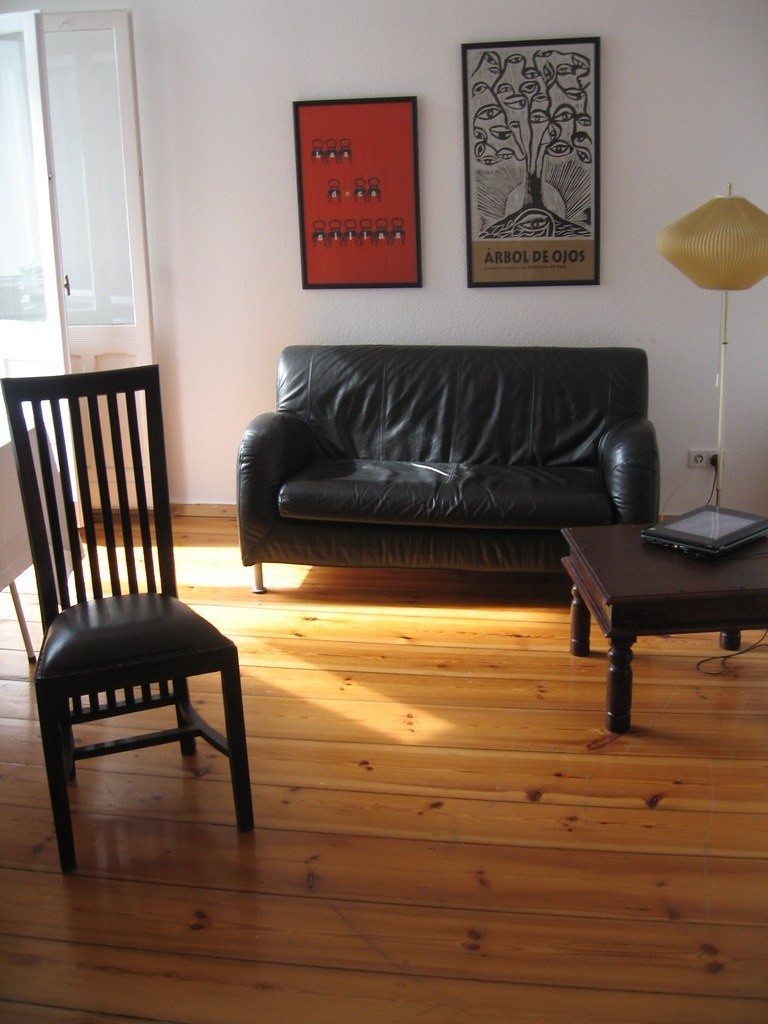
[[0, 415, 86, 666]]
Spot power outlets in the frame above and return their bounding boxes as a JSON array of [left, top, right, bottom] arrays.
[[688, 449, 725, 469]]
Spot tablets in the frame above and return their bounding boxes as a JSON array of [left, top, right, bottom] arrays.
[[640, 504, 768, 550]]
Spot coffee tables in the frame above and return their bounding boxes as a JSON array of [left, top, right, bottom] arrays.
[[560, 526, 768, 735]]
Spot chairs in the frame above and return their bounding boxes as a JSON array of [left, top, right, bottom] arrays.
[[0, 362, 254, 877]]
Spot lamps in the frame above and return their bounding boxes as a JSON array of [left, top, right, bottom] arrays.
[[658, 181, 767, 508]]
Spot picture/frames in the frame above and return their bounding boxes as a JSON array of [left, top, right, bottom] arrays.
[[460, 36, 601, 287], [291, 95, 423, 291]]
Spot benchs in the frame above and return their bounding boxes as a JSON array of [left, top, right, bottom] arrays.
[[235, 344, 658, 599]]
